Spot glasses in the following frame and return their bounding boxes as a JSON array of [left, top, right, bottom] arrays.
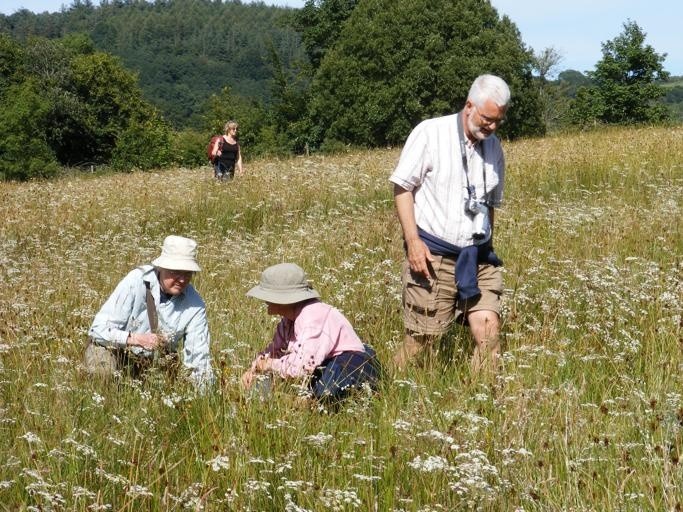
[[476, 108, 507, 126], [168, 270, 196, 279], [232, 128, 238, 130]]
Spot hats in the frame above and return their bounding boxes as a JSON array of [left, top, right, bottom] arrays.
[[151, 236, 201, 272], [246, 263, 321, 305]]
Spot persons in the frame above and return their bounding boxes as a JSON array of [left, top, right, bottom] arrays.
[[389, 74, 512, 378], [85, 235, 215, 395], [238, 262, 385, 407], [211, 118, 244, 181]]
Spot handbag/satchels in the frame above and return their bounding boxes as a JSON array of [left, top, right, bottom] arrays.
[[208, 136, 223, 159]]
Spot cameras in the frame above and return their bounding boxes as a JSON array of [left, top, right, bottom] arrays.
[[464, 198, 492, 240]]
